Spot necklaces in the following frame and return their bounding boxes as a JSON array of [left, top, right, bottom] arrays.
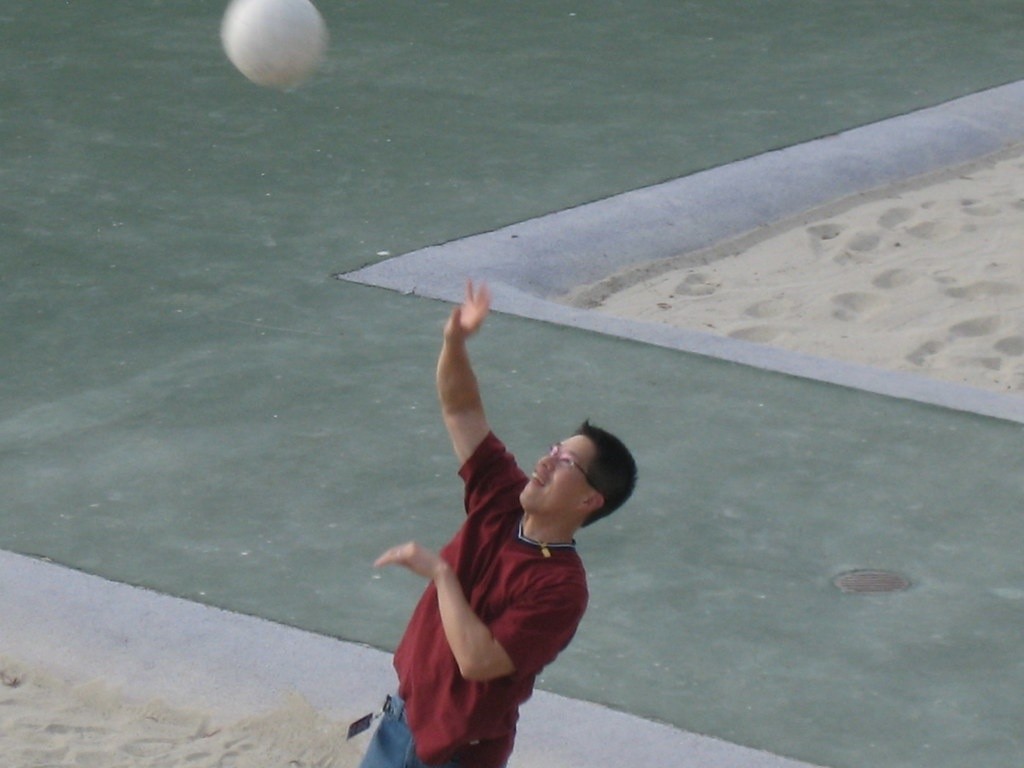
[[536, 539, 551, 557]]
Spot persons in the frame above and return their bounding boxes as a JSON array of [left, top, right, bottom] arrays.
[[359, 279, 638, 768]]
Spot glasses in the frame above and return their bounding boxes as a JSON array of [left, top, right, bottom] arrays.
[[548, 440, 611, 496]]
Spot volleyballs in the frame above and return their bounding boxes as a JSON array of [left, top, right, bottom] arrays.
[[220, 0, 326, 89]]
